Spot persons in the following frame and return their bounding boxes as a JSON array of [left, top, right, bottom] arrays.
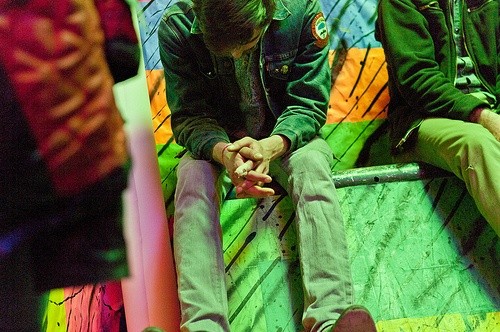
[[157, 0, 377, 332], [375, 0, 500, 245]]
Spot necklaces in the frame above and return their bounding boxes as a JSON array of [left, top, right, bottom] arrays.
[[235, 51, 254, 99]]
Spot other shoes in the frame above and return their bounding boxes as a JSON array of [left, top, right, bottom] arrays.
[[330, 304, 376, 332]]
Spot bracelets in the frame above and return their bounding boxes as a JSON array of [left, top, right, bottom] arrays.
[[221, 143, 233, 164]]
